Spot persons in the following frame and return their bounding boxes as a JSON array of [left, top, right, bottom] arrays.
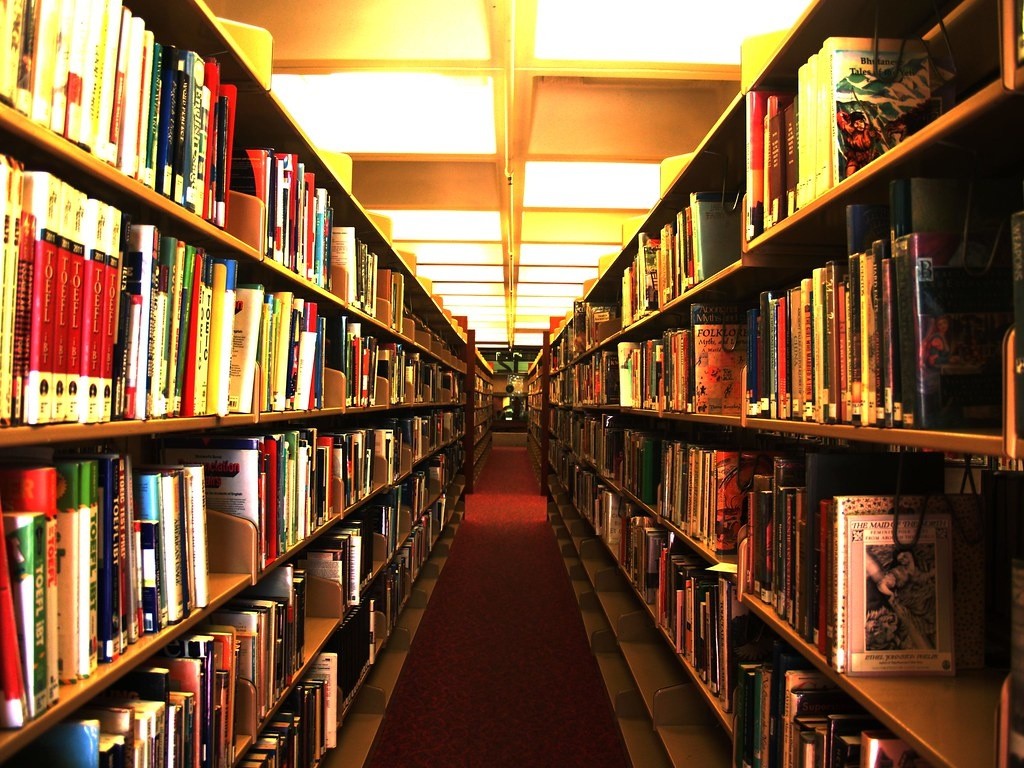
[[496, 397, 522, 421]]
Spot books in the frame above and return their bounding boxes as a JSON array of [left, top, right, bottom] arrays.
[[0, 0, 496, 768], [526, 34, 1024, 768]]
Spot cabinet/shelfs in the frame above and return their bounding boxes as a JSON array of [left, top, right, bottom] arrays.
[[526, 331, 549, 496], [1, 0, 468, 768], [468, 330, 493, 495], [547, 0, 1024, 768]]
